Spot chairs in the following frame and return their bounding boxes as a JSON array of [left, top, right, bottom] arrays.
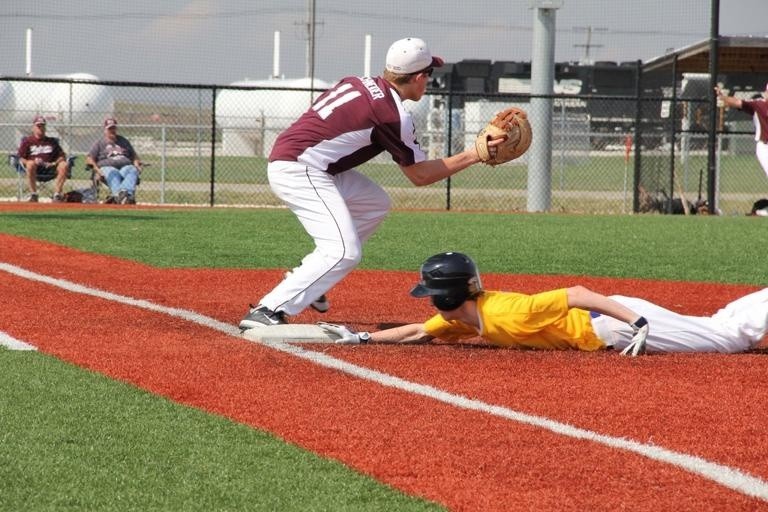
[[79, 160, 152, 204], [7, 154, 78, 203]]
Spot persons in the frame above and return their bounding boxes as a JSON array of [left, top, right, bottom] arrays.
[[714, 82, 768, 217], [17, 116, 68, 202], [238, 37, 505, 330], [85, 119, 143, 205], [317, 252, 768, 356]]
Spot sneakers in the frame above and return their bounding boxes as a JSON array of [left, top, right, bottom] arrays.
[[239, 302, 288, 330], [283, 266, 329, 312], [27, 192, 38, 202], [53, 192, 62, 202], [113, 189, 136, 205]]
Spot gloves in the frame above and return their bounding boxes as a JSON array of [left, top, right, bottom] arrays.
[[317, 321, 362, 345], [620, 316, 649, 357]]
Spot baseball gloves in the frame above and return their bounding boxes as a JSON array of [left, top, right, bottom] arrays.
[[475, 108, 533, 165]]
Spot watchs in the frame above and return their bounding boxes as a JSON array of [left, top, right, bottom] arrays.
[[355, 331, 371, 345]]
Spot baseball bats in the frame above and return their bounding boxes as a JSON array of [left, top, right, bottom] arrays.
[[673, 170, 691, 217]]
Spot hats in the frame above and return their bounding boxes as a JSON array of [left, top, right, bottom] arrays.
[[104, 119, 117, 128], [33, 116, 47, 125], [385, 37, 445, 75]]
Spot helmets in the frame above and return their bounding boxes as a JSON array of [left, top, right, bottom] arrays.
[[409, 252, 481, 311]]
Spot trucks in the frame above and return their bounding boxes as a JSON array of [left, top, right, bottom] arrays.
[[421, 53, 730, 153]]
